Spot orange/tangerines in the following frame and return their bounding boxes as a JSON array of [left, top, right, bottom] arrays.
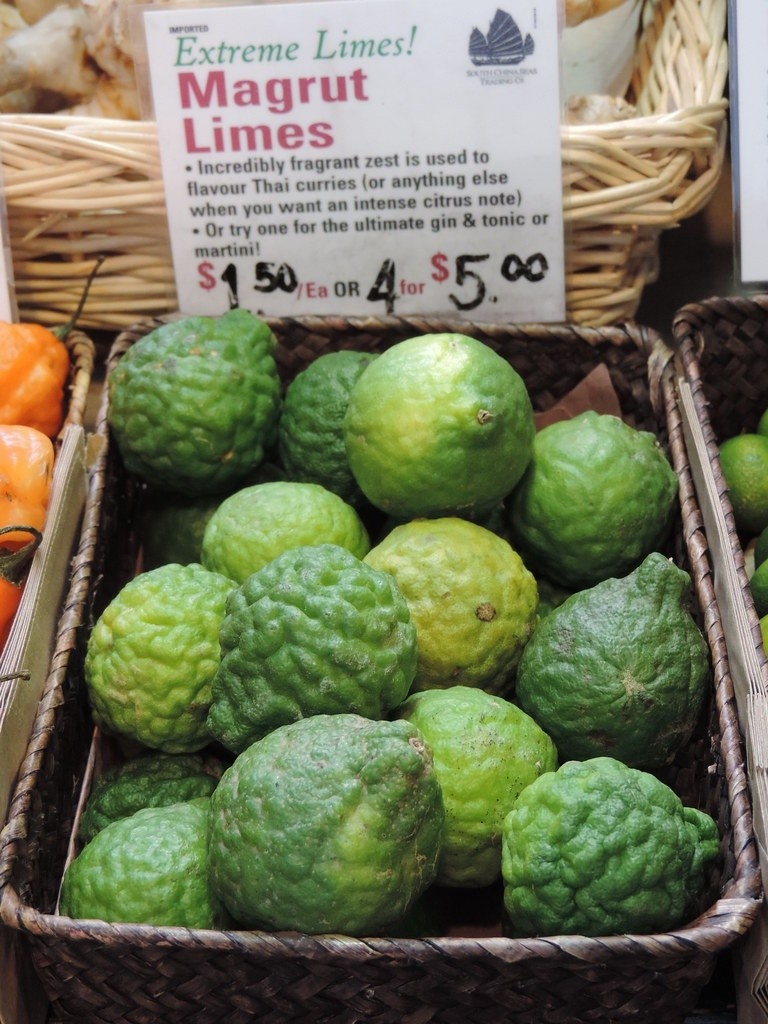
[[715, 400, 768, 659]]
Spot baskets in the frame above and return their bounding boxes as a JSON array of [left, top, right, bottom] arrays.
[[46, 324, 95, 451], [0, 313, 768, 1024], [0, 0, 731, 332], [671, 295, 768, 913]]
